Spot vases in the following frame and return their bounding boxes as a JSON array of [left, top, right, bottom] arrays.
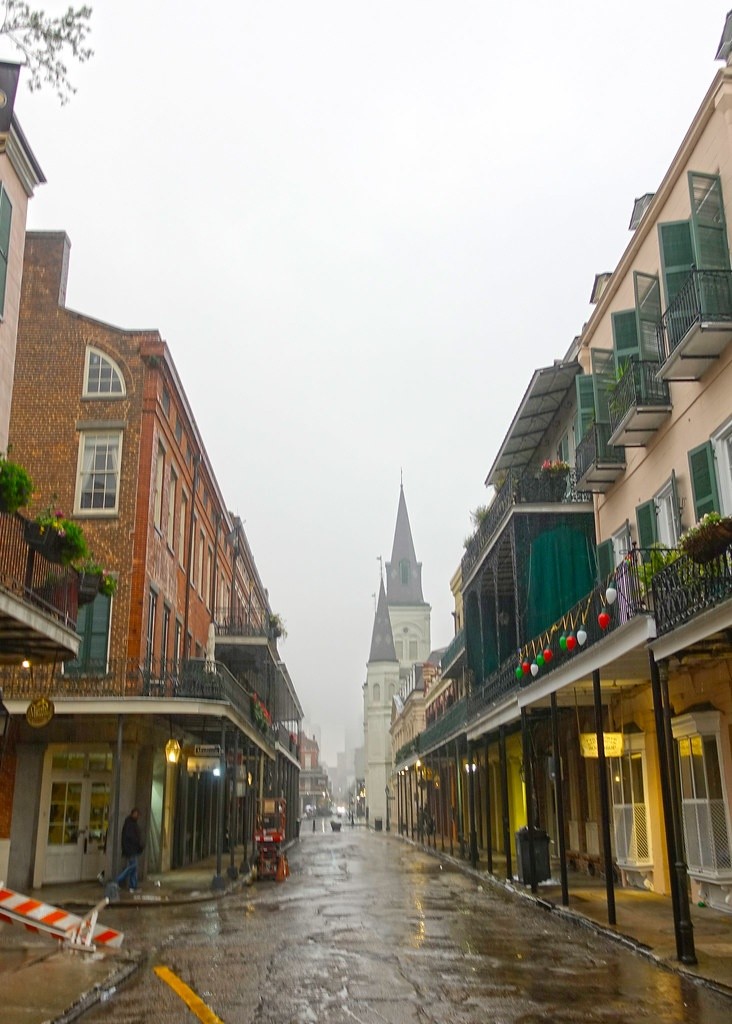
[[78, 573, 100, 606], [270, 622, 278, 628], [24, 522, 70, 565], [275, 630, 281, 636]]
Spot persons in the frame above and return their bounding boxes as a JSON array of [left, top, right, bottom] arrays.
[[114, 807, 144, 893]]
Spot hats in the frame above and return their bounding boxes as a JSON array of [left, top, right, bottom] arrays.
[[132, 807, 141, 816]]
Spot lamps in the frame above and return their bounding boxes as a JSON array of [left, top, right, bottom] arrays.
[[465, 747, 481, 776], [164, 714, 181, 764]]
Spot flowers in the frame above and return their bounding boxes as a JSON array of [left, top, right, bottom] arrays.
[[275, 623, 288, 640], [250, 693, 269, 724], [32, 492, 87, 553], [269, 613, 281, 625], [678, 511, 726, 546], [74, 551, 118, 598], [542, 458, 569, 471]]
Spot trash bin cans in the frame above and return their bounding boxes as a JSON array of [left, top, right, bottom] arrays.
[[375, 816, 382, 831], [515, 832, 551, 886]]
